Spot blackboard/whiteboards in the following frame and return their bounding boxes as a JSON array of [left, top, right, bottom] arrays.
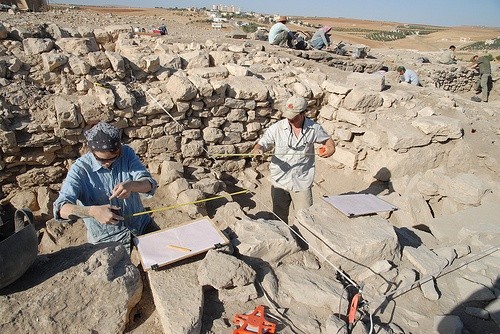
[[134, 216, 230, 271], [322, 193, 398, 218]]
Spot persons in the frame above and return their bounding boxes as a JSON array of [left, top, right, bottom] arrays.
[[134, 26, 145, 33], [439, 45, 458, 64], [53, 123, 157, 261], [0, 190, 21, 224], [397, 66, 419, 85], [472, 55, 491, 102], [376, 66, 389, 75], [309, 25, 331, 49], [268, 16, 295, 47], [249, 96, 336, 226], [157, 22, 167, 35]]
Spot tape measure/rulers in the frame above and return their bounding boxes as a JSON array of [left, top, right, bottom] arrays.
[[212, 147, 326, 159], [111, 189, 250, 224]]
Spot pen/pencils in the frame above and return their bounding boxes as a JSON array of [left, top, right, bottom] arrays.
[[165, 244, 191, 253], [123, 198, 128, 207]]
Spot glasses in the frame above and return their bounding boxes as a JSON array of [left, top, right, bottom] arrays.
[[288, 134, 307, 151], [93, 153, 118, 162]]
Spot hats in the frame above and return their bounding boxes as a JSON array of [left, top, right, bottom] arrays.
[[84, 120, 120, 153], [282, 95, 308, 119], [277, 16, 291, 22]]
